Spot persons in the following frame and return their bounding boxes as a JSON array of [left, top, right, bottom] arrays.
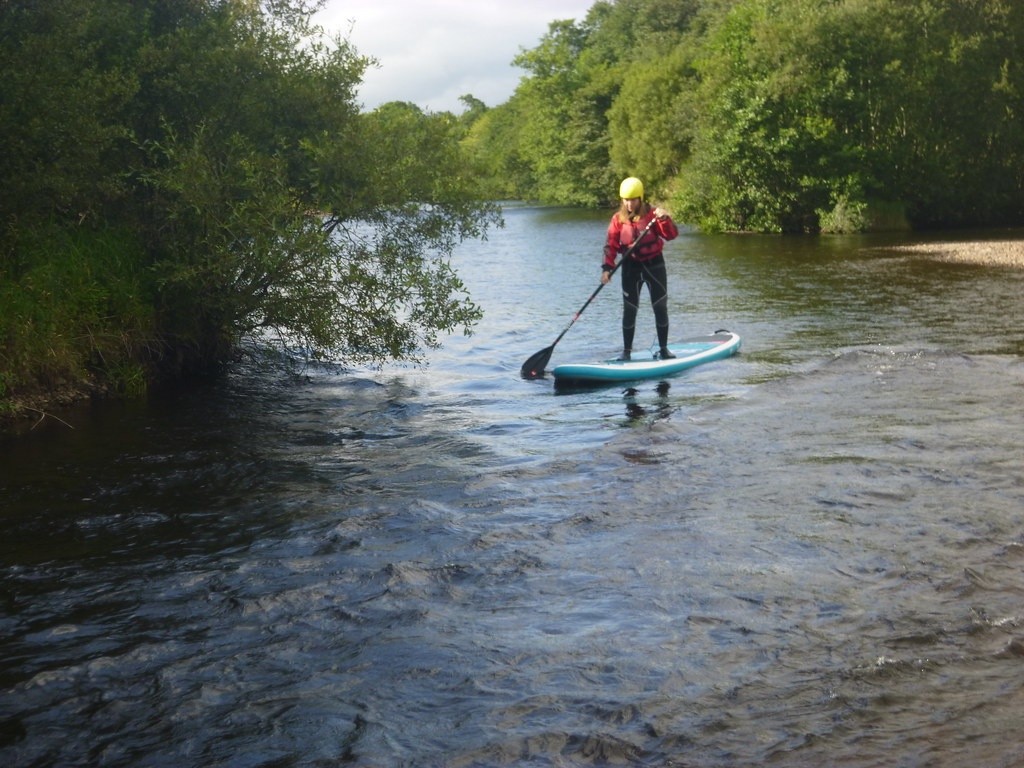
[[602, 177, 678, 361]]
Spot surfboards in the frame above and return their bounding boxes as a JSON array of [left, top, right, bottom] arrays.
[[551, 328, 742, 383]]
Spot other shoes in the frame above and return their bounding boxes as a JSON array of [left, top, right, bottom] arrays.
[[606, 351, 630, 363], [660, 348, 676, 358]]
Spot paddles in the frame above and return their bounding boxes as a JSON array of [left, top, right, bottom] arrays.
[[520, 215, 658, 375]]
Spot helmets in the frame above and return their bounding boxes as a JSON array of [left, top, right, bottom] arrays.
[[620, 177, 645, 201]]
